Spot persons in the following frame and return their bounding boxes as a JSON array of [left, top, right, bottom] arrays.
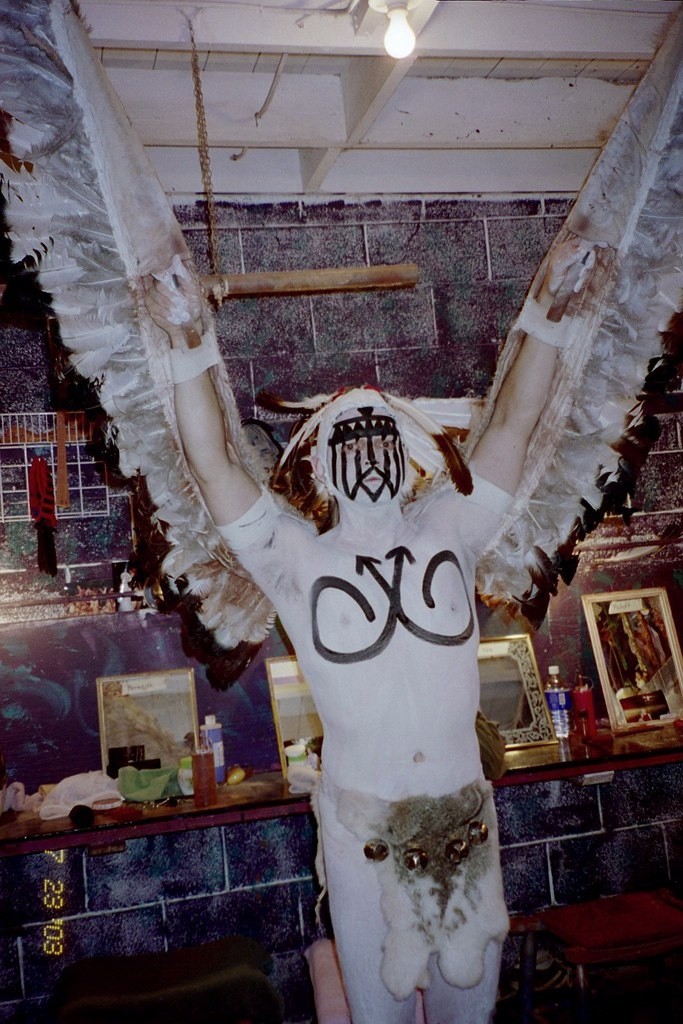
[[141, 236, 609, 1024]]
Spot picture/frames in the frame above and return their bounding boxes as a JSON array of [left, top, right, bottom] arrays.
[[96, 667, 198, 778], [265, 655, 324, 779], [477, 633, 558, 750], [581, 587, 683, 736]]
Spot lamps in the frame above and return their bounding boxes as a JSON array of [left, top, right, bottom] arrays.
[[369, 0, 434, 59]]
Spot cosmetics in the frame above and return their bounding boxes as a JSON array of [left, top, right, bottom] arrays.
[[68, 715, 254, 824]]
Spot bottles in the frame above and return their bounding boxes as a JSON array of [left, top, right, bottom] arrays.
[[544, 665, 570, 737], [199, 715, 226, 780], [572, 674, 598, 738]]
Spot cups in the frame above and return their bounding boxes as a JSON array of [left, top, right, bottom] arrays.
[[191, 753, 218, 809]]
